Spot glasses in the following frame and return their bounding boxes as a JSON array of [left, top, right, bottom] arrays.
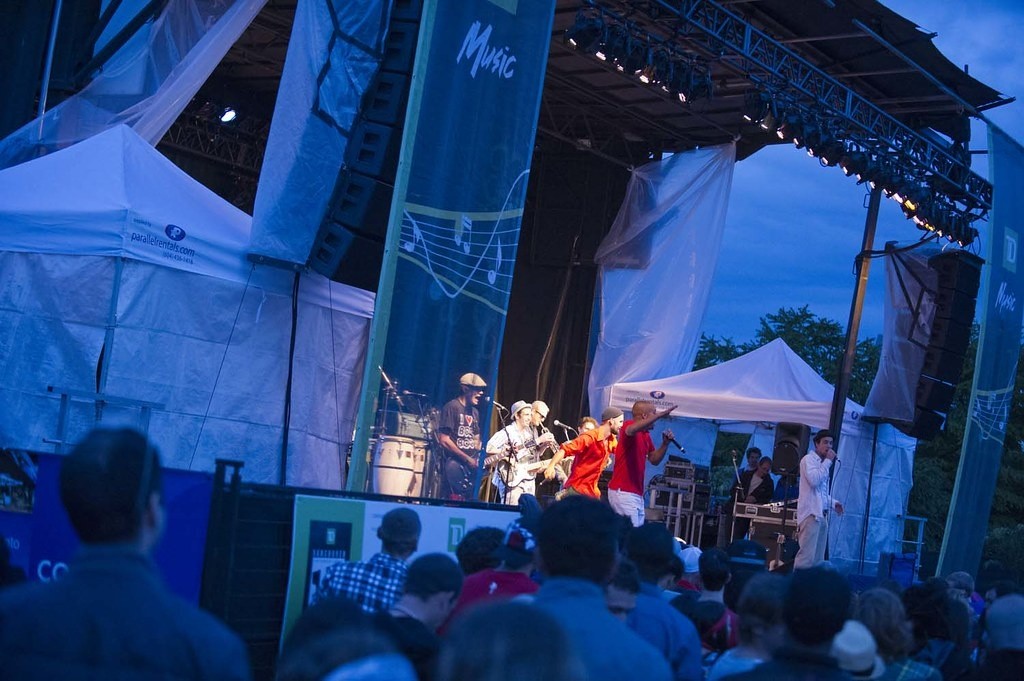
[[535, 410, 546, 419]]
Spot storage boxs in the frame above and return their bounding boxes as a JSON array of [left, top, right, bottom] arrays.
[[650, 461, 711, 512], [734, 502, 798, 524], [748, 520, 799, 576]]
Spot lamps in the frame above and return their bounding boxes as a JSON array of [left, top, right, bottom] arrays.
[[564, 7, 708, 105], [743, 85, 979, 248]]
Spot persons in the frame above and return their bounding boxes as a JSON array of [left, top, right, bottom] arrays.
[[0, 427, 254, 681], [790, 428, 845, 571], [608, 400, 678, 527], [434, 373, 487, 500], [308, 503, 423, 617], [277, 373, 1024, 681]]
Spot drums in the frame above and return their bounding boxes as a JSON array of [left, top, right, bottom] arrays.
[[407, 440, 426, 504], [373, 435, 415, 496]]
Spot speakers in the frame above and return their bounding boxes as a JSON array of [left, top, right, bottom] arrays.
[[770, 421, 811, 476], [308, 0, 454, 290], [887, 249, 985, 441]]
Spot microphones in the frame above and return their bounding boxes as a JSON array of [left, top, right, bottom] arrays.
[[553, 420, 573, 430], [485, 397, 506, 410], [664, 431, 686, 453], [403, 390, 426, 396], [835, 456, 841, 462]]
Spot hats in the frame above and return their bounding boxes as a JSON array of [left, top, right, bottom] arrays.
[[460, 372, 487, 387], [829, 618, 885, 680], [602, 406, 624, 421], [510, 400, 532, 420], [679, 547, 703, 572], [814, 429, 836, 442]]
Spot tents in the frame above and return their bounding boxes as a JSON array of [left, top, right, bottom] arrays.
[[608, 338, 917, 577], [0, 122, 379, 493]]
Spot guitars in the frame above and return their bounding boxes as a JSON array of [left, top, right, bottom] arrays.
[[445, 436, 537, 492], [498, 456, 575, 490]]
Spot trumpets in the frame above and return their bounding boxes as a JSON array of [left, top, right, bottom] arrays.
[[539, 421, 561, 454]]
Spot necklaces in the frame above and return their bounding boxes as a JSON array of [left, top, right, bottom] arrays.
[[390, 602, 435, 635]]
